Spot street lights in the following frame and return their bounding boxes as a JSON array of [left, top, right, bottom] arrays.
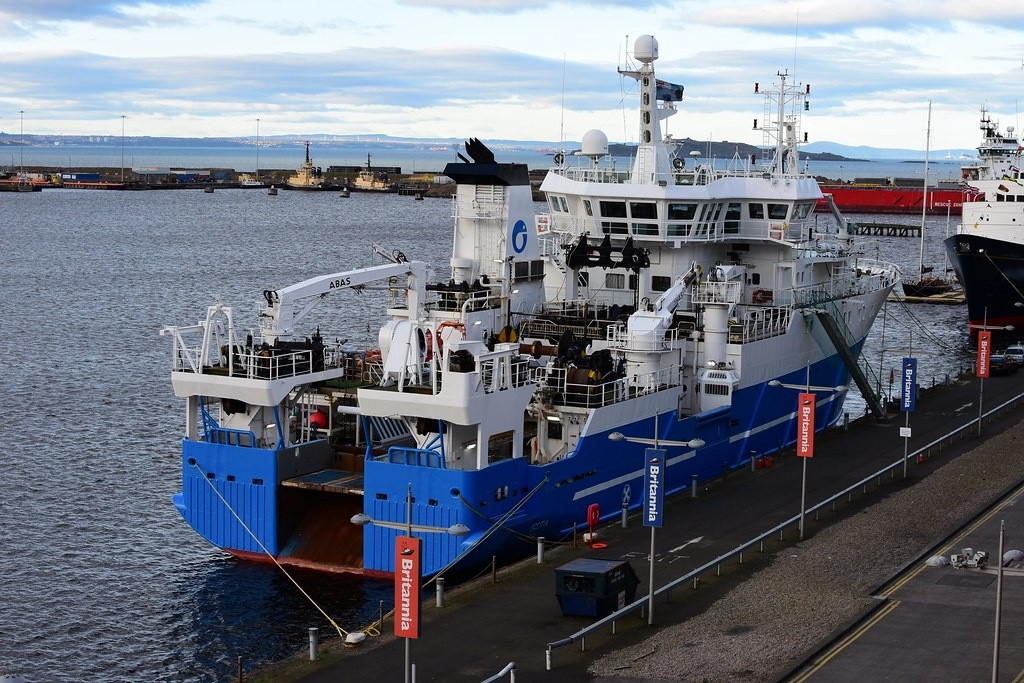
[[120, 115, 127, 184], [20, 110, 24, 174], [255, 119, 260, 182]]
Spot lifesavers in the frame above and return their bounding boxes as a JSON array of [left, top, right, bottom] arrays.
[[539, 220, 548, 232], [770, 226, 781, 240], [755, 290, 767, 304], [592, 543, 607, 549]]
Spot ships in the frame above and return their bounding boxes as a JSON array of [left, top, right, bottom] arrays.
[[943, 99, 1024, 339]]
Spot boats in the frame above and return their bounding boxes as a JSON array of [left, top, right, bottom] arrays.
[[901, 279, 954, 296], [814, 178, 986, 216], [267, 187, 278, 195], [414, 193, 424, 200], [339, 188, 351, 199], [159, 36, 901, 585], [204, 186, 215, 193]]
